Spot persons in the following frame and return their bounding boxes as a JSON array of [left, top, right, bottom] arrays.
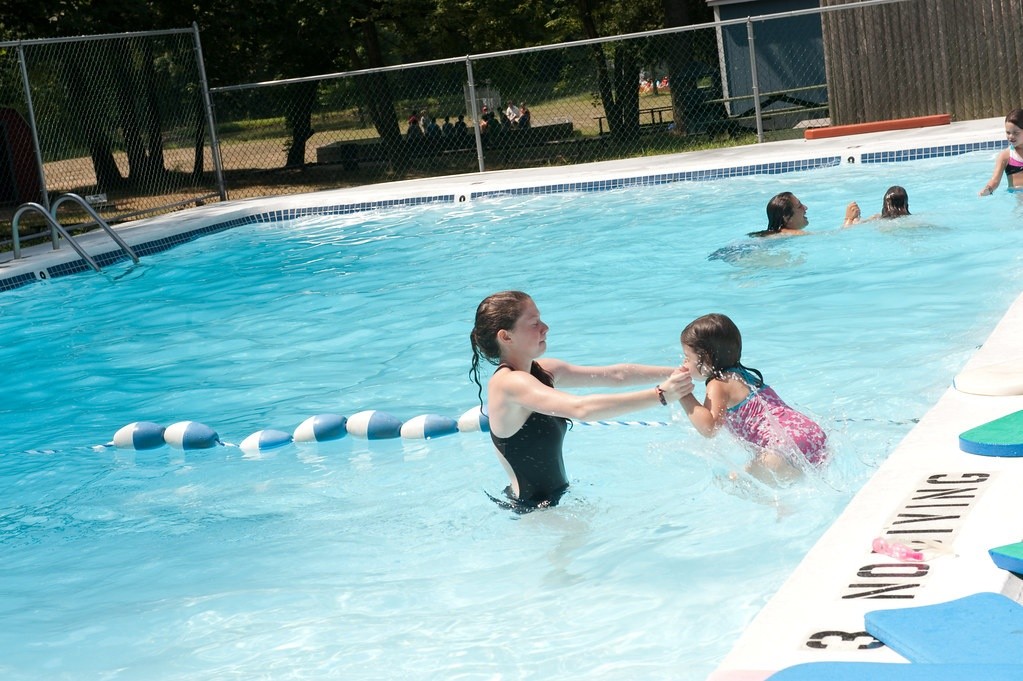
[[981, 109, 1023, 193], [482, 99, 530, 135], [407, 109, 467, 140], [469, 290, 693, 503], [882, 186, 909, 211], [767, 191, 859, 234], [640, 75, 670, 94], [670, 313, 825, 459]]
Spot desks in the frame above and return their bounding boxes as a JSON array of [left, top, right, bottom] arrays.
[[592, 104, 673, 136]]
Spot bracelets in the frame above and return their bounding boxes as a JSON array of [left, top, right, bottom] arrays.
[[655, 385, 667, 405], [987, 185, 993, 194]]
